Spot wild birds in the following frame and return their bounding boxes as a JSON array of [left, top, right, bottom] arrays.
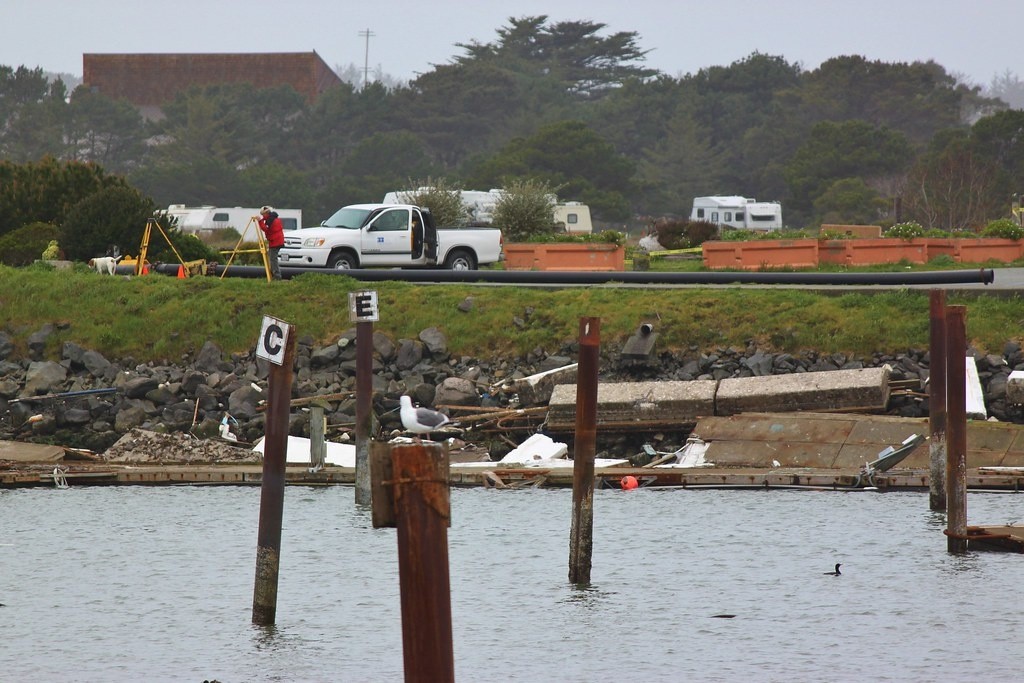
[[822, 561, 846, 577], [398, 394, 452, 445]]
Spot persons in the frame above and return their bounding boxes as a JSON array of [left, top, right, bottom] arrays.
[[255, 206, 285, 280]]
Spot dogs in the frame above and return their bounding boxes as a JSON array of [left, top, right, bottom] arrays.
[[86, 254, 123, 276]]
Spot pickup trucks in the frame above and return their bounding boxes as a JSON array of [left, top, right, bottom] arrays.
[[277, 203, 506, 282]]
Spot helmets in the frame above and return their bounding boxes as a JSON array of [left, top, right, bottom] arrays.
[[260, 206, 271, 214]]
